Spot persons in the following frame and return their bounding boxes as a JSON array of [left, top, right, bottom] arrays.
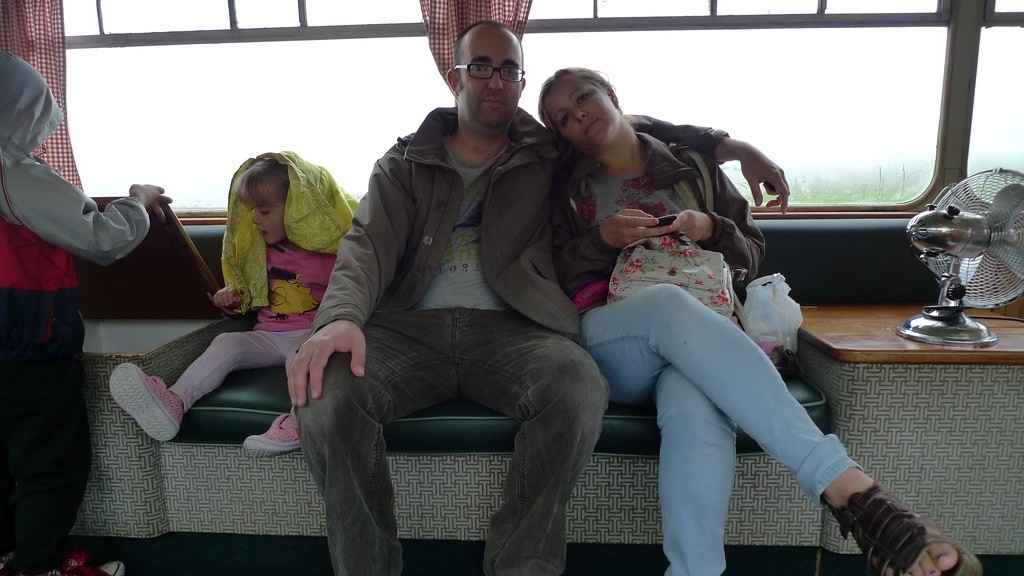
[[0, 49, 172, 576], [288, 20, 982, 576], [109, 152, 359, 457]]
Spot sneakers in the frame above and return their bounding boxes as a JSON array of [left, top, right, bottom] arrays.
[[243, 413, 300, 452], [110, 362, 182, 441]]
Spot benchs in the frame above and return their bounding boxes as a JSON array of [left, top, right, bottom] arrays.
[[164, 361, 830, 576]]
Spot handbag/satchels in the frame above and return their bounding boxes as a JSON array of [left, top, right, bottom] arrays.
[[606, 216, 734, 317]]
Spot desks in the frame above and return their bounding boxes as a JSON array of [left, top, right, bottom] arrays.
[[68, 196, 241, 576], [787, 305, 1024, 576]]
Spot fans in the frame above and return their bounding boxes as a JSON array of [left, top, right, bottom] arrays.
[[897, 168, 1024, 349]]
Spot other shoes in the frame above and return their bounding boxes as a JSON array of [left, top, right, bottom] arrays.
[[45, 552, 125, 576]]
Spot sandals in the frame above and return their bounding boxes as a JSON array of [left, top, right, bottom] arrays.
[[823, 481, 981, 576]]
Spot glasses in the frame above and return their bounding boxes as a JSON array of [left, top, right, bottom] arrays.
[[454, 62, 526, 83]]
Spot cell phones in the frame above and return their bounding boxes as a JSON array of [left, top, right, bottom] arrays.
[[648, 214, 678, 229]]
